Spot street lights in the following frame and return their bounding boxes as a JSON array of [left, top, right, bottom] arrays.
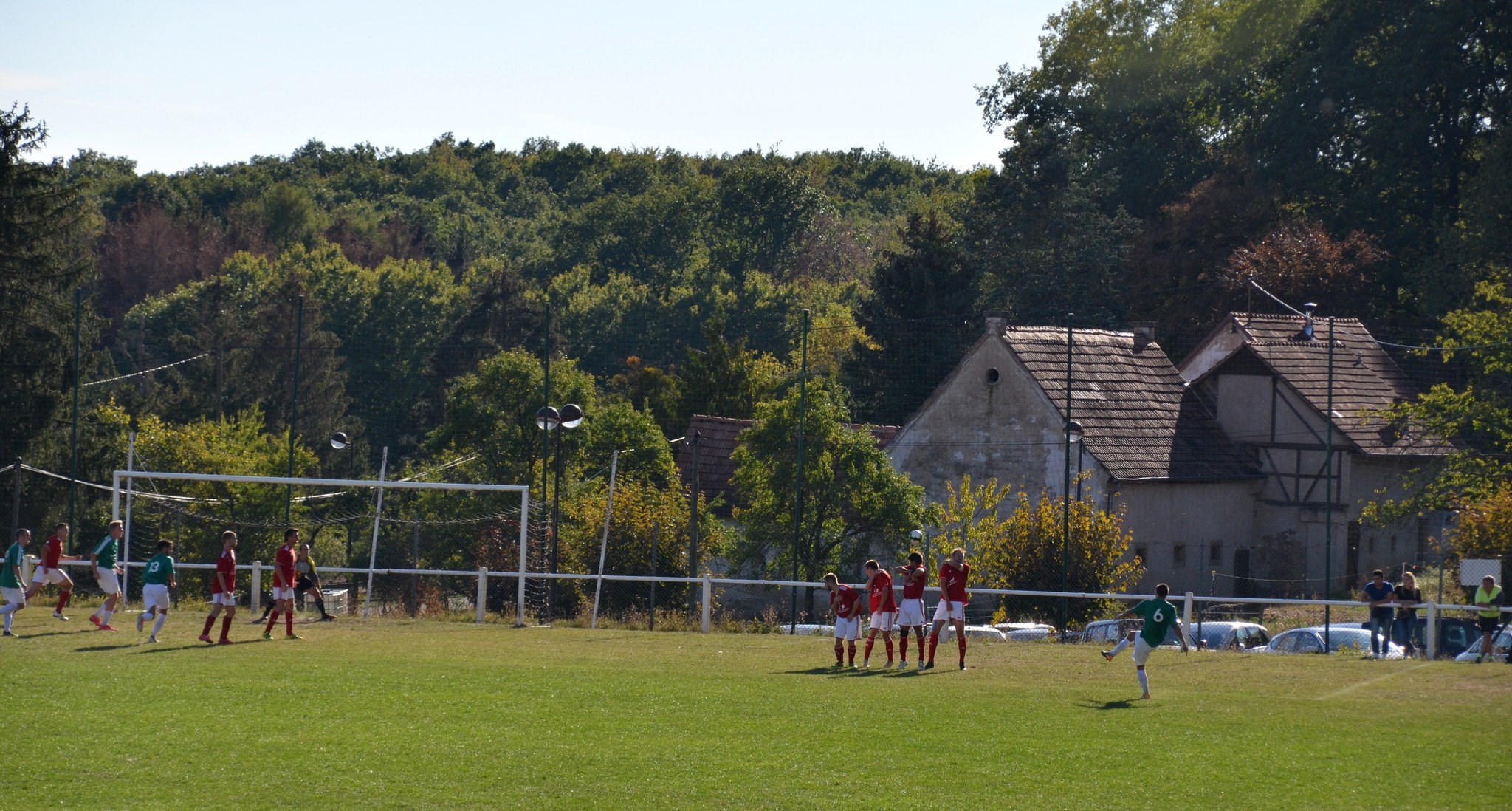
[[329, 431, 354, 568], [535, 403, 585, 620], [1061, 421, 1085, 502]]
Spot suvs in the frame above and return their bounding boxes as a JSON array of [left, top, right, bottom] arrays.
[[1081, 619, 1145, 644]]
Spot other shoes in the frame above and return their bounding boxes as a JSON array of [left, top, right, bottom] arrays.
[[1476, 657, 1482, 663], [959, 662, 966, 670], [1414, 648, 1421, 659], [1382, 654, 1387, 659], [925, 662, 934, 669], [1373, 652, 1379, 658], [1488, 659, 1495, 662]]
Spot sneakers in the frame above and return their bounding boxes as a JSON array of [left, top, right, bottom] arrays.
[[89, 614, 101, 626], [261, 631, 273, 641], [832, 661, 894, 668], [257, 616, 268, 622], [218, 637, 233, 644], [896, 659, 908, 669], [1141, 693, 1150, 699], [99, 624, 116, 631], [918, 660, 925, 670], [3, 630, 19, 636], [285, 634, 303, 639], [322, 614, 335, 619], [198, 634, 213, 643], [1101, 650, 1114, 661], [148, 637, 160, 643], [53, 610, 68, 620], [136, 615, 143, 632]]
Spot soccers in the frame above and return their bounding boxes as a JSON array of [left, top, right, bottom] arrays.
[[909, 530, 923, 544]]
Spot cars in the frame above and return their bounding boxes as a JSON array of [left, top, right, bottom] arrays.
[[1160, 621, 1274, 653], [778, 621, 1087, 645], [1263, 614, 1512, 663]]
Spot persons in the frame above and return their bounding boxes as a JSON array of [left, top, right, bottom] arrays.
[[261, 528, 303, 640], [255, 544, 336, 621], [1101, 583, 1190, 699], [1392, 571, 1422, 659], [90, 520, 126, 631], [136, 539, 177, 643], [1360, 570, 1395, 658], [0, 529, 31, 636], [822, 548, 971, 671], [11, 523, 82, 628], [1475, 575, 1503, 663], [198, 531, 238, 644]]
